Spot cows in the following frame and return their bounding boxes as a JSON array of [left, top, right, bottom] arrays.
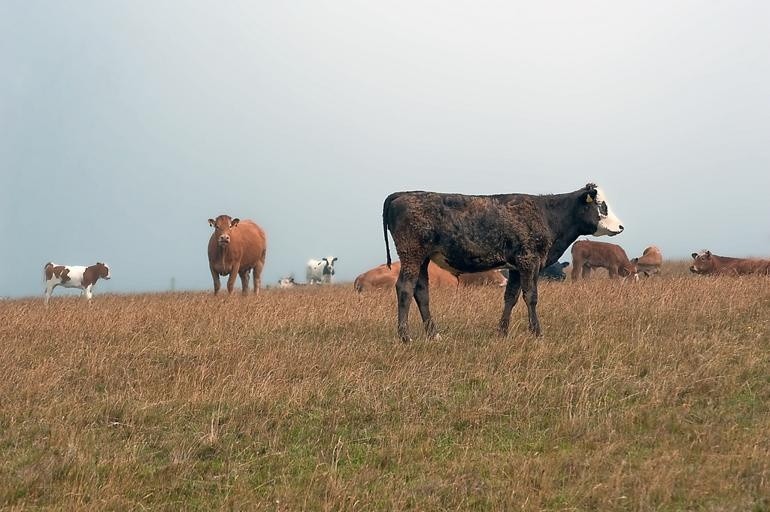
[[383, 182, 624, 341], [304, 256, 337, 285], [499, 261, 569, 282], [42, 261, 111, 305], [207, 215, 266, 295], [631, 245, 663, 276], [278, 276, 298, 288], [690, 251, 769, 277], [354, 261, 507, 292], [571, 241, 639, 281]]
